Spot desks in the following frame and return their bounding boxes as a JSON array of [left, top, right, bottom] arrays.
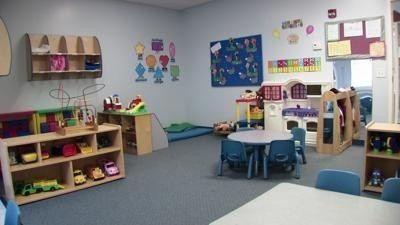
[[227, 129, 294, 176], [208, 183, 400, 225]]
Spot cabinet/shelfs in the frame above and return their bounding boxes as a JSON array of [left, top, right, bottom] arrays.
[[25, 32, 102, 80], [361, 121, 399, 193], [97, 111, 153, 156], [0, 106, 125, 207]]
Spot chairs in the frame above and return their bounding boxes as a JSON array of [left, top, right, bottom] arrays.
[[315, 168, 361, 195], [358, 96, 372, 125], [291, 127, 308, 163], [217, 138, 255, 179], [236, 127, 255, 154], [262, 139, 303, 179], [382, 177, 400, 203], [4, 200, 22, 225]]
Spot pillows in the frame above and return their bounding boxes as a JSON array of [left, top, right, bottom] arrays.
[[165, 123, 194, 132]]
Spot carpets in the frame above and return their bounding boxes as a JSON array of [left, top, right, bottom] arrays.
[[162, 126, 213, 143]]
